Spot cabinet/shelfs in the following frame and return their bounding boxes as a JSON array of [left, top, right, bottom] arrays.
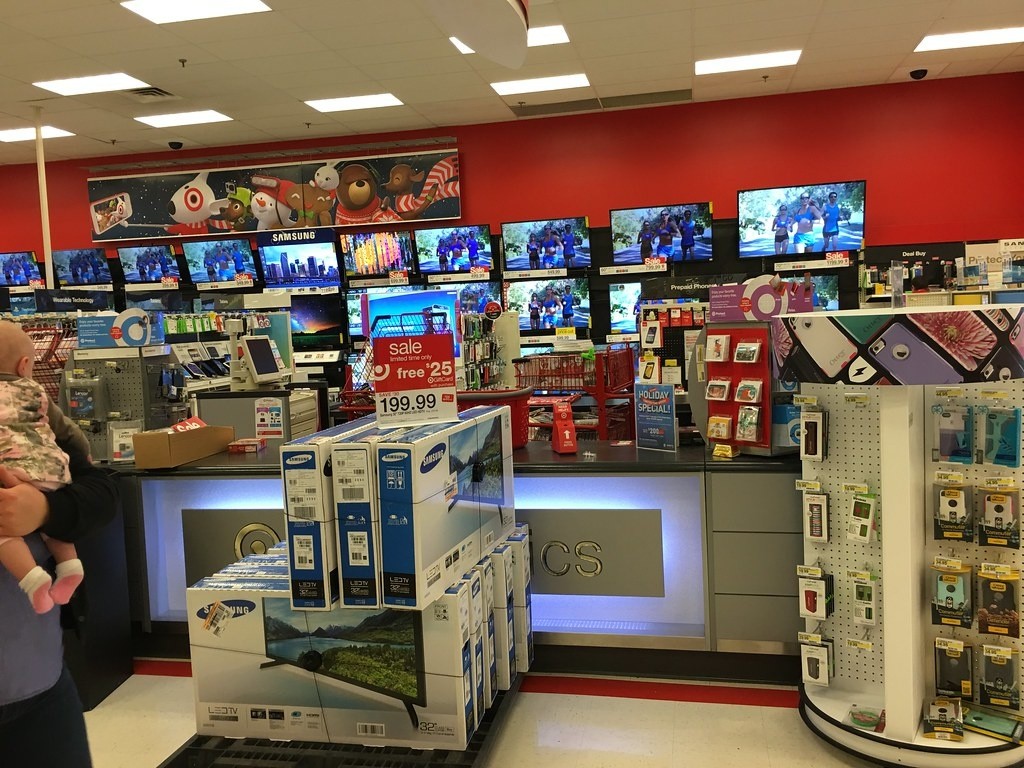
[[512, 342, 635, 445]]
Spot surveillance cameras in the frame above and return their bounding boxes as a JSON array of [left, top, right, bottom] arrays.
[[168, 142, 183, 150], [910, 69, 927, 80]]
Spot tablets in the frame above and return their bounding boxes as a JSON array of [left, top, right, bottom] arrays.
[[179, 364, 191, 378], [184, 362, 206, 376], [187, 349, 202, 362], [199, 362, 216, 374], [211, 359, 230, 374], [207, 347, 219, 357]]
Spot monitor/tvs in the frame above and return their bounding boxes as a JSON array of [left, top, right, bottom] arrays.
[[0, 216, 591, 359], [778, 276, 841, 311], [445, 415, 506, 525], [609, 202, 714, 266], [737, 180, 866, 260], [260, 597, 427, 728], [608, 283, 699, 335], [239, 335, 282, 384]]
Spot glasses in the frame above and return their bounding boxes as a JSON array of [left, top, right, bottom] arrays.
[[781, 209, 787, 211], [830, 196, 837, 198], [685, 214, 691, 216], [646, 225, 649, 226], [801, 197, 810, 199], [451, 239, 456, 241], [216, 246, 222, 247], [661, 214, 669, 216], [545, 228, 551, 230], [566, 227, 570, 229]]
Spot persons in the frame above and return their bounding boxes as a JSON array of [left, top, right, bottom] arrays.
[[526, 225, 578, 267], [771, 192, 843, 254], [437, 231, 480, 272], [633, 295, 692, 333], [0, 440, 96, 768], [812, 283, 819, 305], [637, 210, 697, 261], [204, 242, 246, 281], [136, 249, 170, 281], [461, 290, 488, 314], [69, 252, 100, 283], [0, 319, 92, 614], [2, 255, 32, 285], [528, 285, 576, 329]]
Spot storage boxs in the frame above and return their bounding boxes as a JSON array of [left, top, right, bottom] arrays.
[[226, 438, 267, 453], [186, 404, 535, 752], [132, 425, 235, 470]]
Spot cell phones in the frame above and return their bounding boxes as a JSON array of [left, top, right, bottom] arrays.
[[789, 306, 1024, 385], [646, 327, 656, 344], [643, 363, 654, 378]]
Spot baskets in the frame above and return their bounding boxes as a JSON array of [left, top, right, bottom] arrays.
[[456, 385, 534, 450]]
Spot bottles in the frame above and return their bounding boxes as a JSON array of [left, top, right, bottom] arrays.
[[164, 314, 212, 334]]
